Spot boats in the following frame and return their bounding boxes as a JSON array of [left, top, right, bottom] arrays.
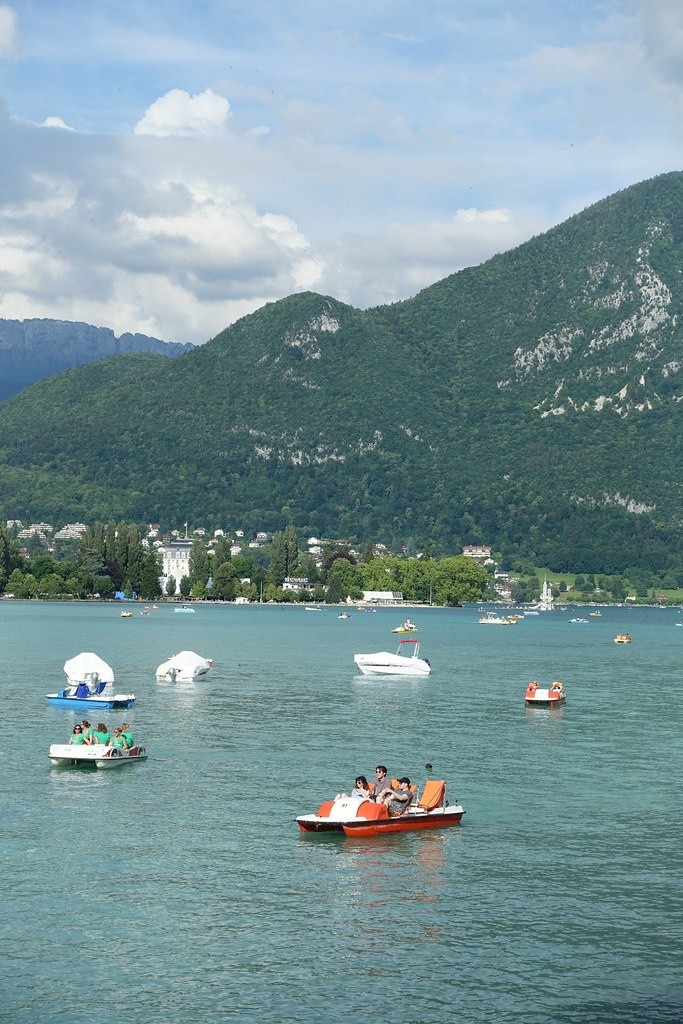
[[588, 610, 603, 617], [48, 744, 148, 770], [391, 618, 418, 634], [174, 604, 195, 614], [478, 611, 539, 625], [524, 681, 567, 704], [568, 617, 589, 624], [305, 606, 322, 611], [338, 612, 353, 619], [155, 651, 213, 682], [613, 634, 632, 644], [120, 604, 160, 617], [45, 650, 136, 710], [354, 640, 432, 675], [293, 780, 466, 839]]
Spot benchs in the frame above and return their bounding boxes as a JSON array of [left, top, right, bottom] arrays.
[[367, 780, 418, 817]]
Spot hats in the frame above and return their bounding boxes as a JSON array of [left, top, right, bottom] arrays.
[[397, 777, 410, 784]]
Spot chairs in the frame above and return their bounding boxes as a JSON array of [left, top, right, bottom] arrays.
[[69, 683, 89, 697], [89, 681, 107, 697], [409, 780, 447, 815]]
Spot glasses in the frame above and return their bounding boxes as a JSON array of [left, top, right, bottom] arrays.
[[375, 771, 381, 773], [74, 728, 80, 730], [81, 724, 84, 726], [357, 782, 361, 784], [114, 731, 117, 733]]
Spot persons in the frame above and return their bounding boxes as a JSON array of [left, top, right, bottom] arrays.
[[352, 776, 374, 804], [108, 727, 128, 749], [595, 609, 600, 614], [403, 618, 416, 631], [340, 611, 348, 617], [121, 723, 134, 749], [576, 617, 583, 622], [626, 634, 631, 640], [527, 681, 539, 691], [181, 606, 186, 608], [376, 777, 413, 815], [616, 632, 622, 640], [93, 723, 110, 744], [369, 766, 395, 804], [553, 684, 563, 693], [69, 725, 94, 745], [81, 721, 95, 744]]
